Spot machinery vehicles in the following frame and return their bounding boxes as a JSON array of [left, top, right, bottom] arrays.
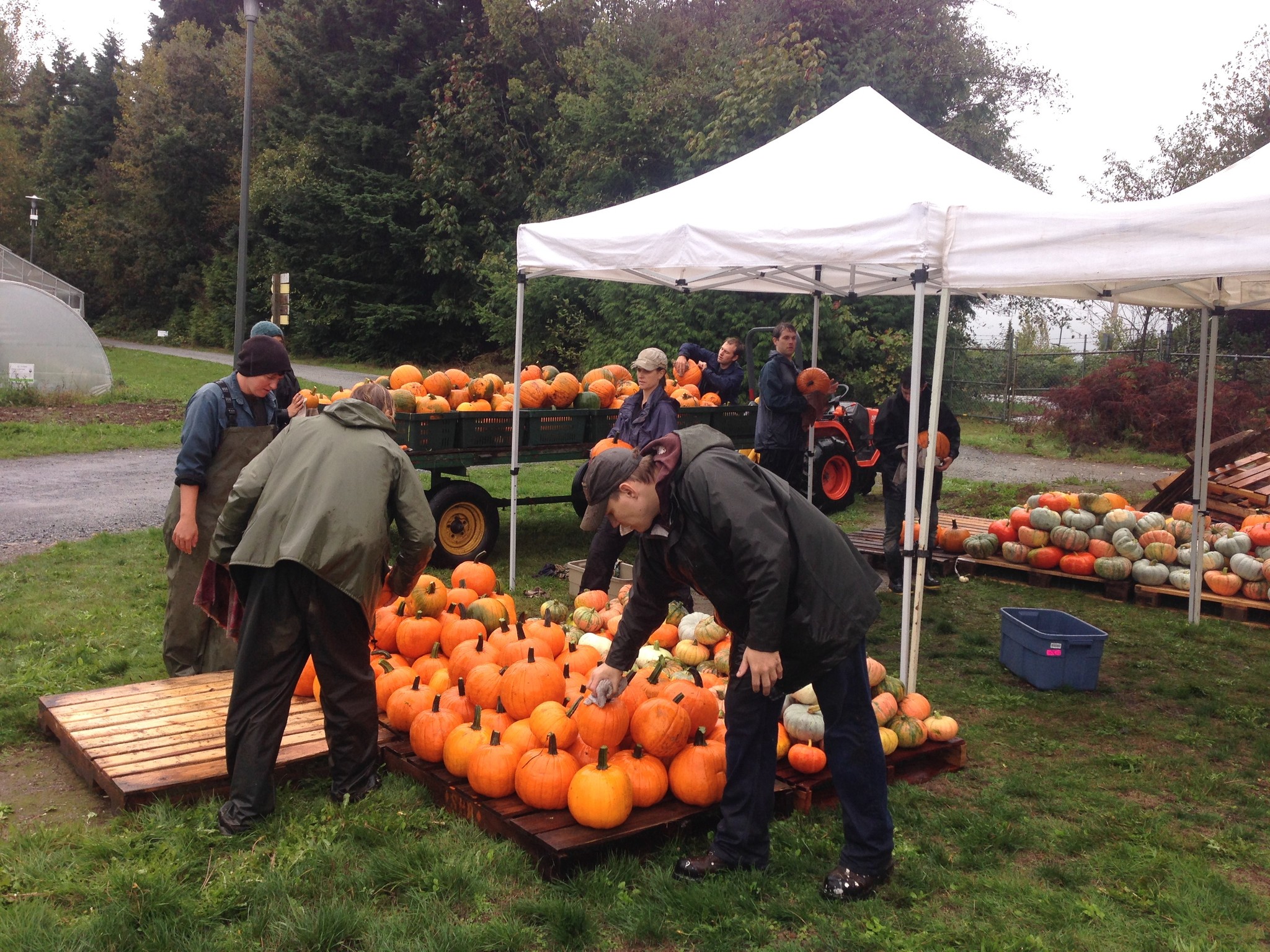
[[318, 326, 896, 572]]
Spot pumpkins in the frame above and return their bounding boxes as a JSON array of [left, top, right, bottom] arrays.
[[796, 368, 830, 395], [291, 550, 959, 828], [898, 492, 1270, 603], [297, 357, 760, 462], [918, 430, 950, 463]]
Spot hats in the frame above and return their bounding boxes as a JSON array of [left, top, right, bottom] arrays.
[[250, 321, 284, 341], [630, 347, 668, 372], [580, 447, 643, 532], [237, 337, 292, 377]]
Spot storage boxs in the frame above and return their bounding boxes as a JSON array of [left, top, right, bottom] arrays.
[[1000, 607, 1109, 689], [567, 559, 633, 600]]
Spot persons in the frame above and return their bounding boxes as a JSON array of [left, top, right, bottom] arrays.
[[573, 323, 960, 904], [161, 335, 292, 678], [206, 384, 437, 833], [250, 321, 305, 435]]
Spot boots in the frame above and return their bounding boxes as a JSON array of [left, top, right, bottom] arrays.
[[915, 539, 941, 589], [885, 553, 914, 595]]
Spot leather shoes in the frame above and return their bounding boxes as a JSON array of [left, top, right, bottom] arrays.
[[823, 858, 894, 899], [673, 850, 733, 881]]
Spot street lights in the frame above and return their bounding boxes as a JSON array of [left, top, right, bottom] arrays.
[[24, 195, 44, 264]]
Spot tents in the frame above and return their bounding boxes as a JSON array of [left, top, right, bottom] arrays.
[[508, 89, 1270, 695]]
